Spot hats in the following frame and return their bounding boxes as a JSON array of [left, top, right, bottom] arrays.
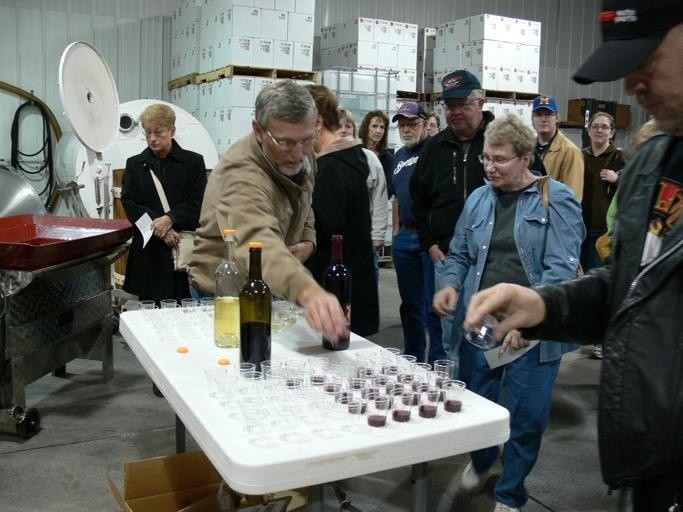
[[532, 95, 559, 113], [440, 70, 482, 102], [571, 0, 683, 87], [391, 102, 426, 123]]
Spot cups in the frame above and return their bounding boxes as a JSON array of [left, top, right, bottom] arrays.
[[207, 347, 468, 428], [464, 314, 497, 350], [136, 295, 212, 349], [271, 297, 310, 338]]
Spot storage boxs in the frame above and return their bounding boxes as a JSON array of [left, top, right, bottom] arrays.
[[314, 15, 544, 152], [107, 452, 315, 511], [566, 97, 630, 129], [165, 1, 315, 158]]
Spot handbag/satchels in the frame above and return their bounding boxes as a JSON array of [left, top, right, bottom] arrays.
[[172, 230, 196, 273]]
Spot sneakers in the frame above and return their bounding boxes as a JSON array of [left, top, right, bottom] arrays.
[[589, 343, 604, 359], [492, 502, 520, 512], [460, 452, 500, 492]]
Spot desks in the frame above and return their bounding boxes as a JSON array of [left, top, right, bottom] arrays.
[[120, 302, 511, 510]]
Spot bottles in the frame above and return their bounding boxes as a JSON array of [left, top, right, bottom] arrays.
[[241, 241, 271, 380], [213, 228, 242, 350], [322, 235, 354, 351]]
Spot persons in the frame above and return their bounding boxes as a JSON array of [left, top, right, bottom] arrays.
[[461, 0, 681, 512], [357, 111, 395, 199], [597, 117, 665, 361], [433, 114, 589, 512], [121, 104, 207, 399], [386, 101, 448, 368], [299, 84, 382, 338], [333, 107, 387, 284], [407, 70, 546, 383], [580, 112, 623, 361], [186, 78, 350, 341], [530, 94, 584, 207], [421, 110, 440, 137]]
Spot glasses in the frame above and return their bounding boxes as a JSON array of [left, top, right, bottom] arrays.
[[395, 121, 424, 130], [266, 127, 320, 152], [477, 152, 522, 164]]
